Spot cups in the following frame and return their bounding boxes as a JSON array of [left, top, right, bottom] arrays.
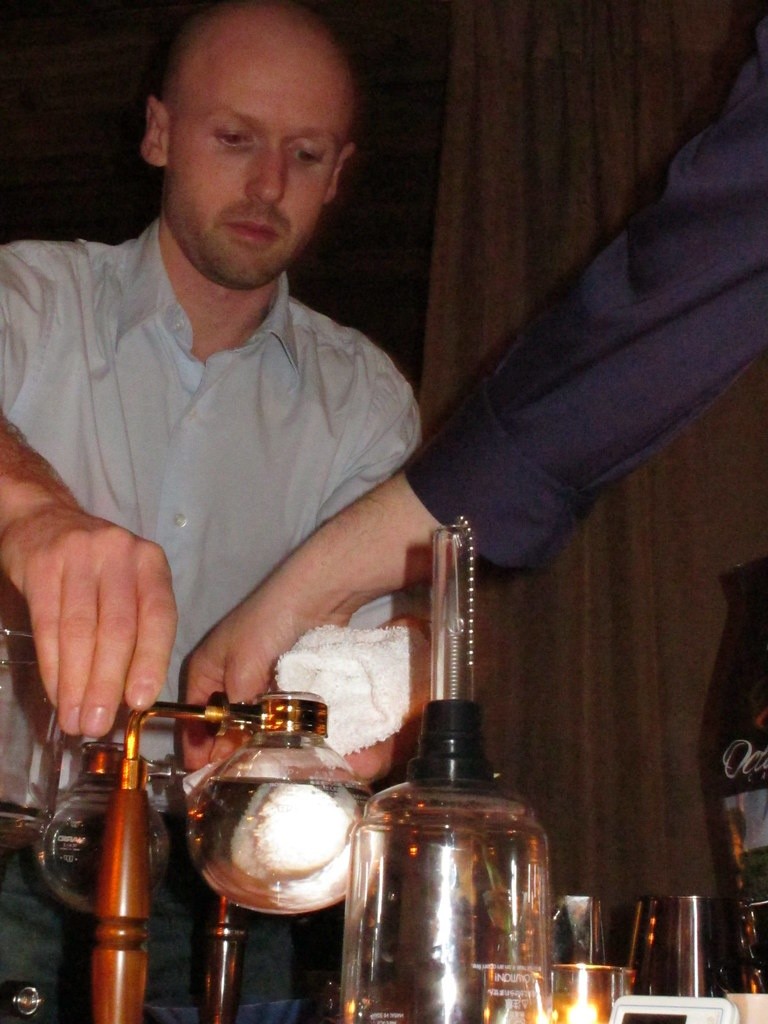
[[626, 896, 744, 997], [483, 964, 547, 1024]]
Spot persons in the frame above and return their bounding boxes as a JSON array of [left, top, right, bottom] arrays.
[[0, 1, 433, 1024], [175, 16, 767, 773]]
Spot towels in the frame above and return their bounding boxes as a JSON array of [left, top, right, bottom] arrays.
[[180, 624, 431, 914]]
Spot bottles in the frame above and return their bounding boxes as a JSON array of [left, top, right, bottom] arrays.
[[551, 964, 636, 1024], [699, 555, 768, 993], [341, 700, 552, 1024]]
